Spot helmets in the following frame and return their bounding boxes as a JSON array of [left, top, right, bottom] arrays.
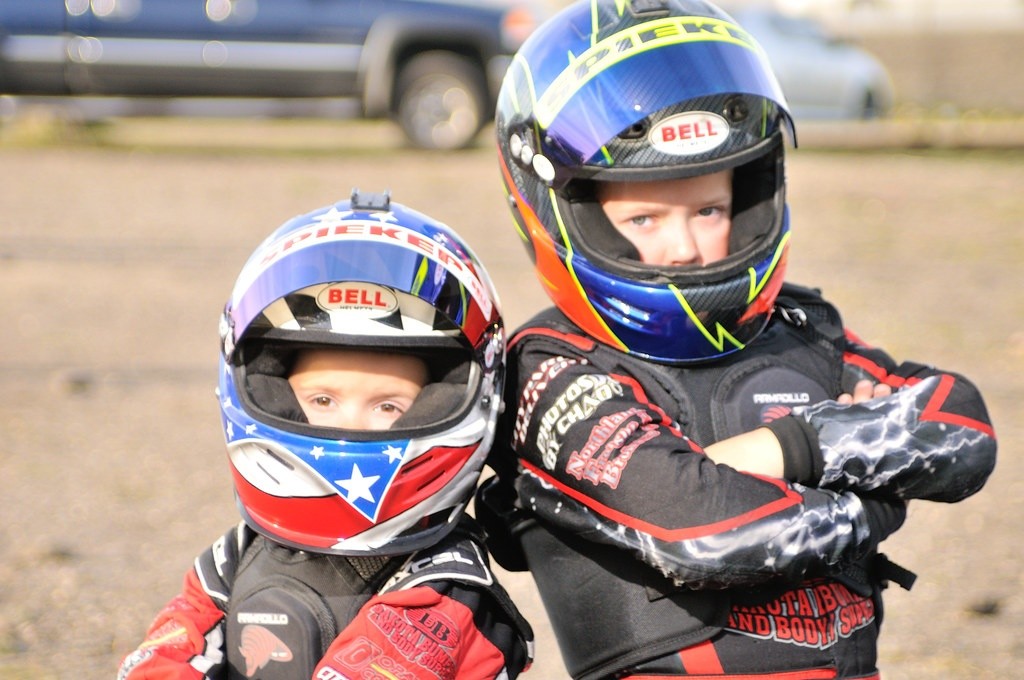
[[494, 0, 791, 363], [215, 189, 506, 554]]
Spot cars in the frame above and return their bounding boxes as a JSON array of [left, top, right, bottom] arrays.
[[0, 0, 549, 151], [724, 1, 896, 122]]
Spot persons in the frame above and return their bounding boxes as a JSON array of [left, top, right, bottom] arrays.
[[111, 187, 534, 679], [475, 0, 1000, 680]]
